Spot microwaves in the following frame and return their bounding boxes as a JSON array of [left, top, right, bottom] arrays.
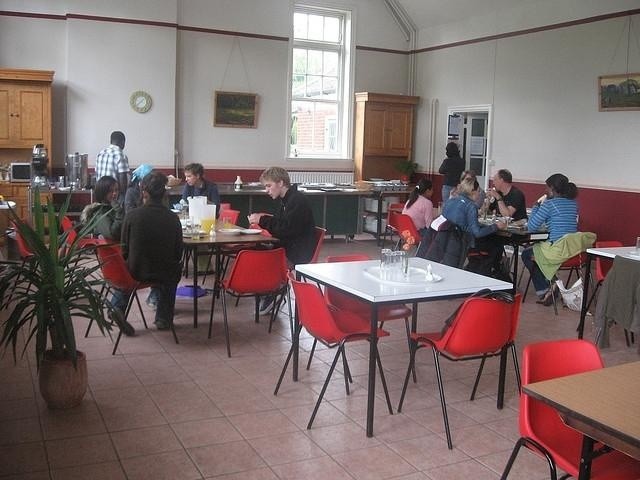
[[10, 162, 32, 183]]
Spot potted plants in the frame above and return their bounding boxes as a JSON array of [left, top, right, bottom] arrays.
[[0, 161, 138, 409], [396, 161, 420, 185]]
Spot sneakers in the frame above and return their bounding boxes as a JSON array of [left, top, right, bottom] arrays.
[[543, 283, 559, 306], [259, 294, 281, 315], [156, 317, 169, 329], [108, 307, 135, 336]]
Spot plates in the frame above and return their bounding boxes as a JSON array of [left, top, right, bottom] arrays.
[[218, 229, 240, 235], [240, 229, 262, 235]]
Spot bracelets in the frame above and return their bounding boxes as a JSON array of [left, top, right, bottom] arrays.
[[496, 198, 504, 202]]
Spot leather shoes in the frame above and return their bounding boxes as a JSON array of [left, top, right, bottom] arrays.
[[146, 297, 158, 311]]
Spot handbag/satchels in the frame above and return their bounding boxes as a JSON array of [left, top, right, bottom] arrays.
[[555, 277, 583, 312]]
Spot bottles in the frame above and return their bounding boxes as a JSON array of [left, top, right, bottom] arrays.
[[234, 175, 243, 192], [425, 264, 433, 281]]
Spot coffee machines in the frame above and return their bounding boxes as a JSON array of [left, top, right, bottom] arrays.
[[64, 151, 89, 191]]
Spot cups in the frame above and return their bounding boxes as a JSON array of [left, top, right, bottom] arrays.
[[172, 218, 214, 240], [379, 248, 409, 284], [223, 217, 232, 229]]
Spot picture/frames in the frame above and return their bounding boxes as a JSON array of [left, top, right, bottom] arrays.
[[211, 90, 258, 128], [599, 71, 640, 112]]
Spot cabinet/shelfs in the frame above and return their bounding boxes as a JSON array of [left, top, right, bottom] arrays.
[[355, 93, 422, 156], [0, 69, 55, 148], [0, 181, 50, 229]]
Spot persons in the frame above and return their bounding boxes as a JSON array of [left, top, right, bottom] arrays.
[[398, 177, 435, 237], [441, 173, 506, 274], [79, 176, 161, 310], [180, 162, 222, 276], [479, 170, 534, 225], [436, 142, 469, 201], [245, 165, 320, 317], [94, 129, 133, 190], [106, 167, 188, 337], [122, 163, 154, 214], [460, 170, 485, 208], [523, 173, 582, 307]]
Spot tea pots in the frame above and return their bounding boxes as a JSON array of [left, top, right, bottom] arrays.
[[187, 196, 208, 219]]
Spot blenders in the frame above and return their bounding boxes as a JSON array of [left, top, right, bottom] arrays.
[[31, 144, 49, 186]]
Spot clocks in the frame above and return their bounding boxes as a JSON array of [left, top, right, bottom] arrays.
[[131, 90, 152, 114]]
